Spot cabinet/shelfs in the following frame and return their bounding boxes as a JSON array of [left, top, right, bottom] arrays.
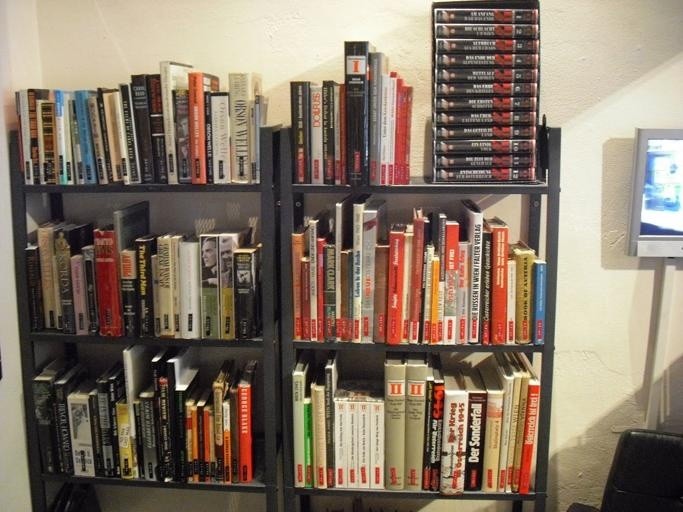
[[8, 120, 284, 511], [277, 124, 561, 512]]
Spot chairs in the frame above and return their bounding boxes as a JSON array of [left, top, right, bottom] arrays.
[[564, 428, 682, 512]]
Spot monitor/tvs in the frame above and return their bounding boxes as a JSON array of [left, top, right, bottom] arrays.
[[626, 129, 683, 259]]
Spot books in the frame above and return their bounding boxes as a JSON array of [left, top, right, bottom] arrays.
[[291, 194, 546, 346], [25, 201, 262, 340], [32, 341, 258, 483], [292, 351, 543, 496], [15, 61, 267, 183], [433, 0, 542, 183], [290, 41, 413, 186]]
